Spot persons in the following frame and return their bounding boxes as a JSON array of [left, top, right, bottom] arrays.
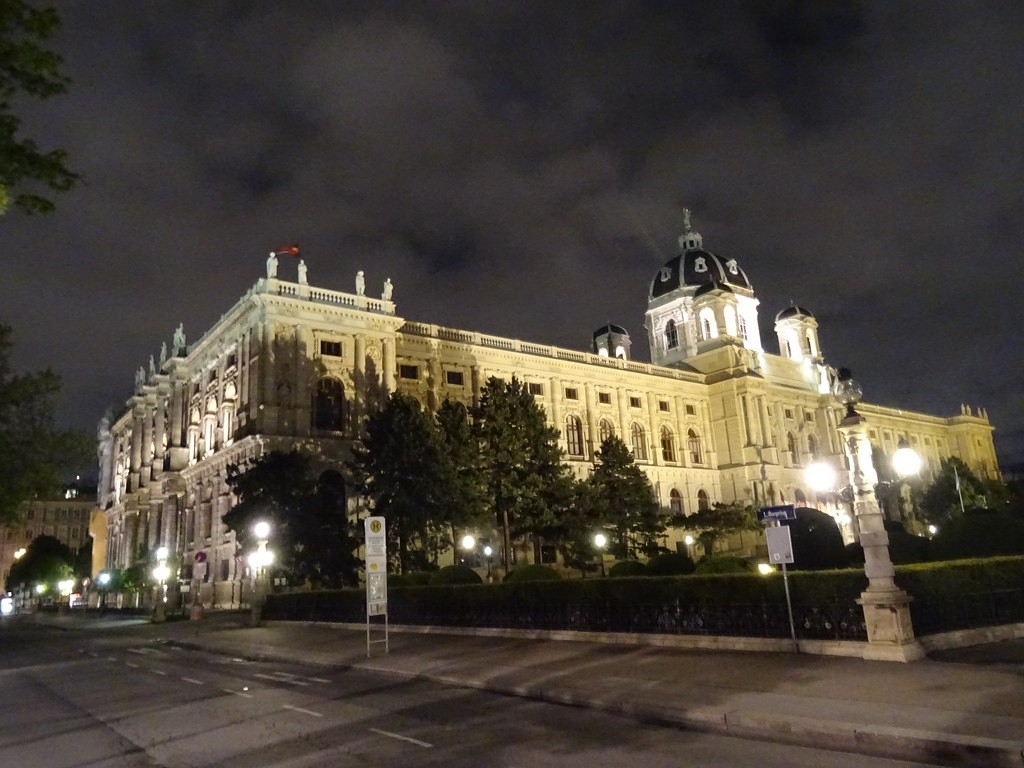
[[383, 278, 393, 300], [977, 407, 987, 418], [961, 404, 971, 416], [136, 324, 185, 388], [356, 271, 365, 296], [298, 260, 307, 284], [267, 252, 278, 278], [191, 603, 203, 637]]
